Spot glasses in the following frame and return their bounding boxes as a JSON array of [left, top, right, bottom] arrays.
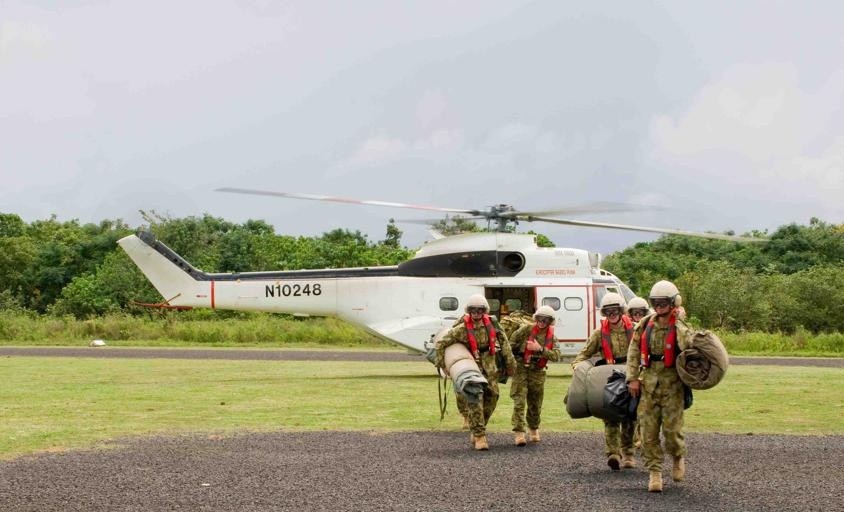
[[651, 297, 672, 307], [469, 306, 487, 314], [536, 314, 553, 324], [604, 307, 620, 315], [631, 308, 646, 316]]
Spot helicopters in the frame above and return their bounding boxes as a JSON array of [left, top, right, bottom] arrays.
[[114, 185, 774, 385]]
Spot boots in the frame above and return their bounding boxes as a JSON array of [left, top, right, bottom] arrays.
[[669, 459, 686, 482], [515, 429, 526, 445], [607, 453, 635, 470], [474, 435, 490, 450], [530, 428, 539, 441], [648, 469, 663, 491]]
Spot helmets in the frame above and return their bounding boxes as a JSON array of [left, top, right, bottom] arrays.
[[464, 294, 490, 314], [532, 305, 558, 326], [600, 293, 626, 315], [650, 280, 681, 307], [626, 297, 648, 314]]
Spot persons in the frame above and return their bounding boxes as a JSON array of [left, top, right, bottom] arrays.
[[627, 299, 649, 447], [572, 293, 639, 470], [508, 305, 561, 446], [436, 295, 517, 450], [627, 281, 694, 492]]
